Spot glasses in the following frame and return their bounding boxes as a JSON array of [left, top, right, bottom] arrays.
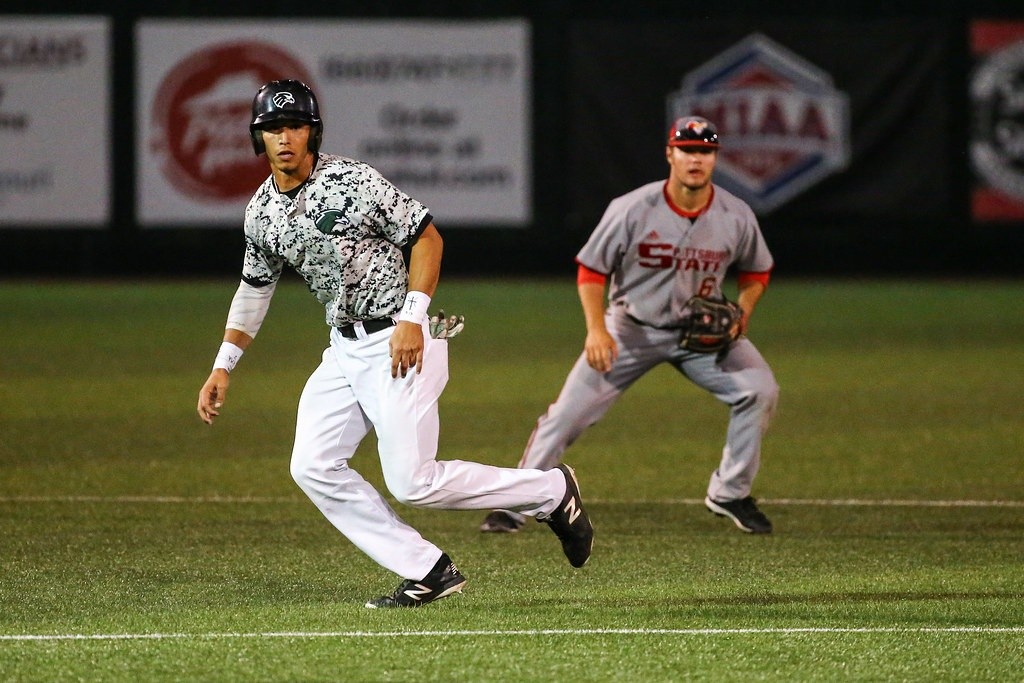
[[670, 129, 719, 143]]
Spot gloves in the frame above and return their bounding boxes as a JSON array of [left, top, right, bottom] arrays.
[[429, 308, 465, 339]]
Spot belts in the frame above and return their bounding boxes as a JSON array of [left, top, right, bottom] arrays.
[[337, 317, 396, 338]]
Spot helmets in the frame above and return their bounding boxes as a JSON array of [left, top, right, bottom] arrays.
[[248, 80, 323, 157]]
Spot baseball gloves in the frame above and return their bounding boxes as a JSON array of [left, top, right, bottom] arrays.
[[677, 295, 748, 365]]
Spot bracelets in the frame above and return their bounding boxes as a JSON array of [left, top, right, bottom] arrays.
[[213, 342, 243, 375], [399, 290, 431, 325]]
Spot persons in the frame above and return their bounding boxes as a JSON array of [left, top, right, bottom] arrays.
[[482, 117, 779, 533], [198, 78, 594, 610]]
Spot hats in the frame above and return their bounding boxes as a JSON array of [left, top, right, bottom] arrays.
[[668, 115, 720, 149]]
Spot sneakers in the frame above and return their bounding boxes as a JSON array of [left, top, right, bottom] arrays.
[[705, 494, 773, 534], [536, 463, 594, 568], [480, 510, 518, 533], [365, 553, 466, 609]]
